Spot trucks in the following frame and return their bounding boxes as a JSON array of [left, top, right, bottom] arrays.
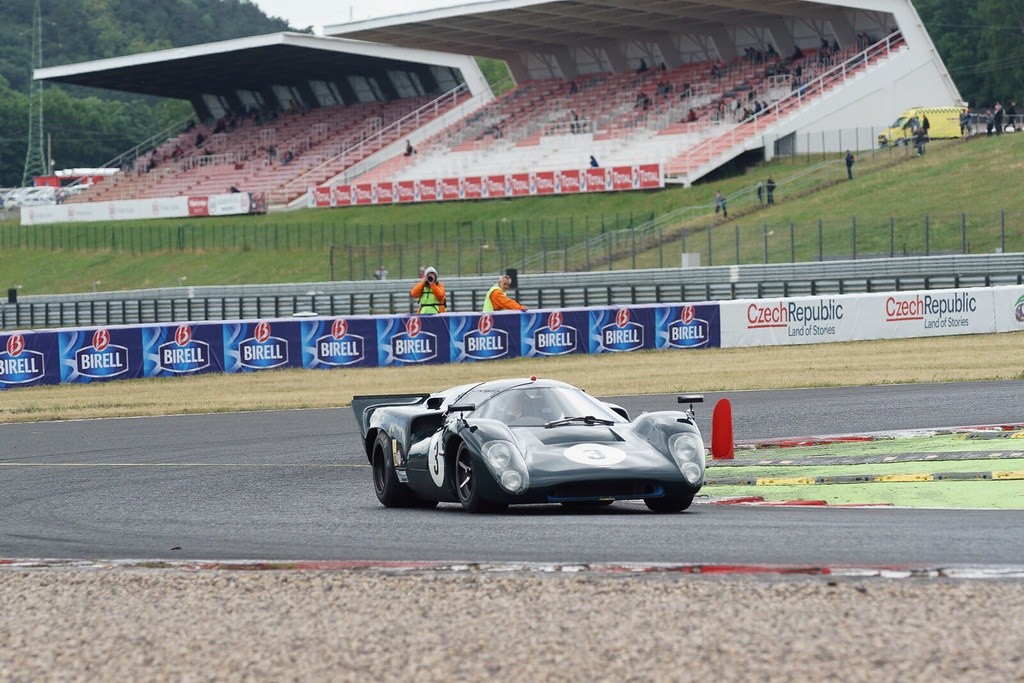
[[877, 106, 968, 147]]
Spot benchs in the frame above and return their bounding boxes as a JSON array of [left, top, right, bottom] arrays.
[[61, 36, 905, 204]]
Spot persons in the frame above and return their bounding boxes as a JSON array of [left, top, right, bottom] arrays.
[[911, 115, 930, 157], [713, 189, 720, 215], [144, 98, 296, 192], [409, 266, 446, 314], [374, 266, 387, 280], [720, 193, 727, 216], [495, 394, 539, 425], [402, 26, 899, 169], [417, 265, 425, 278], [757, 180, 765, 204], [845, 149, 854, 179], [958, 99, 1018, 136], [482, 275, 528, 313], [766, 175, 776, 204]]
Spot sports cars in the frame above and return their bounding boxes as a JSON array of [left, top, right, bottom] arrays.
[[350, 373, 706, 514]]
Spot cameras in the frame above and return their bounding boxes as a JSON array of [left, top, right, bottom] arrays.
[[427, 275, 433, 282]]
